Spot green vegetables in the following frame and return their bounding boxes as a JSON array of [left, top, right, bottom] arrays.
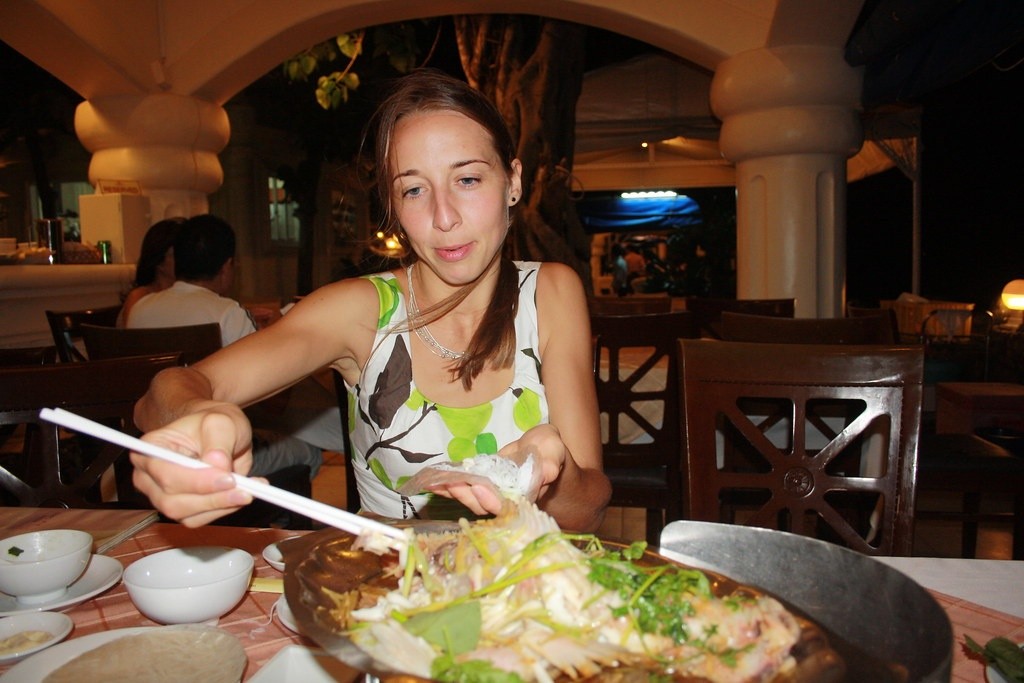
[[963, 633, 1024, 683], [390, 531, 755, 683]]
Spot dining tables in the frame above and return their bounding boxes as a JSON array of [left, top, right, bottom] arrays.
[[0, 506, 917, 681]]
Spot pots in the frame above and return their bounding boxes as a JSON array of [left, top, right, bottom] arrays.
[[274, 520, 954, 682]]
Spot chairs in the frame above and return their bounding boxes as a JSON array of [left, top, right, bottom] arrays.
[[46, 295, 1023, 681]]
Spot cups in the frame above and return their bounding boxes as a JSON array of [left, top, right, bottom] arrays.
[[0, 238, 37, 255], [36, 219, 64, 263]]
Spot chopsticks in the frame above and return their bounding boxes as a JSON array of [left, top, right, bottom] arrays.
[[38, 407, 406, 551]]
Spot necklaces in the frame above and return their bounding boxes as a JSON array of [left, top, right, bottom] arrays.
[[406, 263, 469, 360]]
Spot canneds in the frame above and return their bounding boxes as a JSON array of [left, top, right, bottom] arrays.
[[97, 240, 113, 264]]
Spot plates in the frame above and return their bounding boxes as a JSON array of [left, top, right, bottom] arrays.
[[985, 643, 1024, 683], [261, 543, 285, 572], [0, 612, 73, 661], [0, 627, 162, 683], [277, 591, 302, 636], [0, 554, 123, 617]]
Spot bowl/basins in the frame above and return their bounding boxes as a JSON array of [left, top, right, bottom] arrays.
[[0, 530, 93, 604], [122, 546, 254, 625]]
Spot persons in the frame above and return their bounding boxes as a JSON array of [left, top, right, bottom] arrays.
[[675, 229, 727, 310], [129, 65, 612, 546], [125, 213, 322, 530], [612, 237, 645, 295], [117, 217, 188, 328]]
